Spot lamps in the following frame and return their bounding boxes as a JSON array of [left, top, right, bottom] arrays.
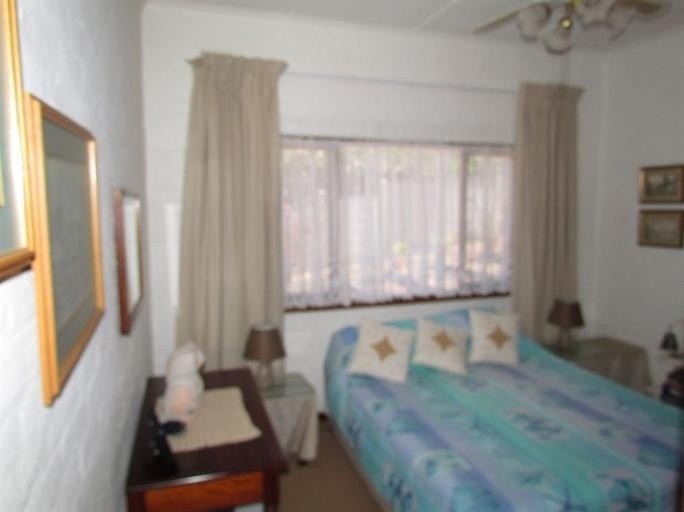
[[241, 321, 285, 388], [545, 297, 585, 359], [515, 0, 664, 53]]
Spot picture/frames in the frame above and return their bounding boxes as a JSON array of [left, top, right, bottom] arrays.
[[110, 185, 143, 337], [24, 88, 106, 408], [636, 163, 684, 249], [0, 1, 36, 280]]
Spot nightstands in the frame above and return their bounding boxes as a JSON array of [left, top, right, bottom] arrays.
[[553, 339, 652, 398], [247, 373, 317, 471]]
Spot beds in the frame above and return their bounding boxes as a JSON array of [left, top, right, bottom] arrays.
[[324, 310, 684, 512]]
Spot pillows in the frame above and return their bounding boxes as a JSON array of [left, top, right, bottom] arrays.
[[344, 306, 522, 384]]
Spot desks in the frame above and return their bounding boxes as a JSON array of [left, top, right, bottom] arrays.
[[125, 367, 287, 511]]
